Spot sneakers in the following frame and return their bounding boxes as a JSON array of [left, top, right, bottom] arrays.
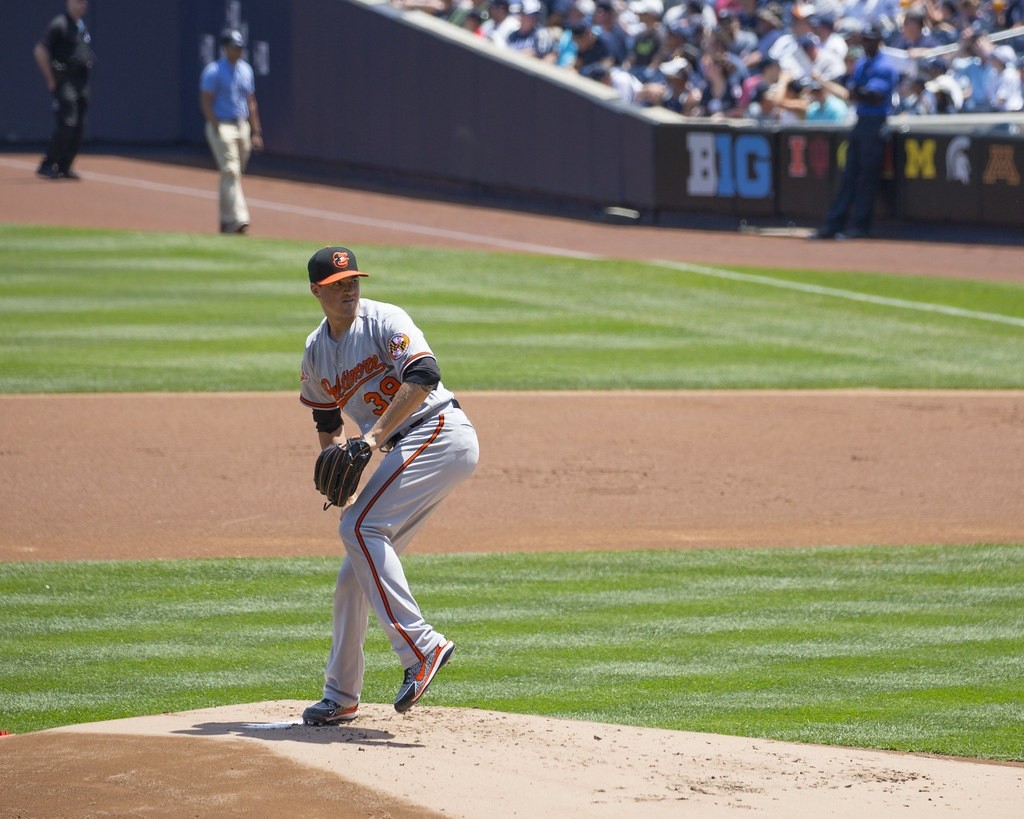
[[302, 698, 359, 725], [394, 637, 455, 715]]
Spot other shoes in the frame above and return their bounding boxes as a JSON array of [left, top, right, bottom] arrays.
[[839, 227, 869, 238], [36, 161, 64, 179], [62, 170, 81, 180], [809, 230, 834, 241], [238, 224, 250, 235]]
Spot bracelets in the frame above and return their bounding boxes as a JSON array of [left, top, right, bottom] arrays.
[[252, 128, 264, 136]]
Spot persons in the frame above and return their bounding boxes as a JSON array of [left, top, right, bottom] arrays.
[[199, 27, 264, 234], [300, 247, 479, 728], [390, 0, 1024, 241], [33, 0, 95, 179]]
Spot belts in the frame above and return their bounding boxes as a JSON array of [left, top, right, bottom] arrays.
[[384, 400, 461, 454]]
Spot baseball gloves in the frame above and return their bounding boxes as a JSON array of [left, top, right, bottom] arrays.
[[312, 438, 373, 509]]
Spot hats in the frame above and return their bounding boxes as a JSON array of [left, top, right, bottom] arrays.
[[217, 29, 247, 47], [308, 245, 370, 285], [859, 26, 881, 40], [628, 0, 662, 14]]
